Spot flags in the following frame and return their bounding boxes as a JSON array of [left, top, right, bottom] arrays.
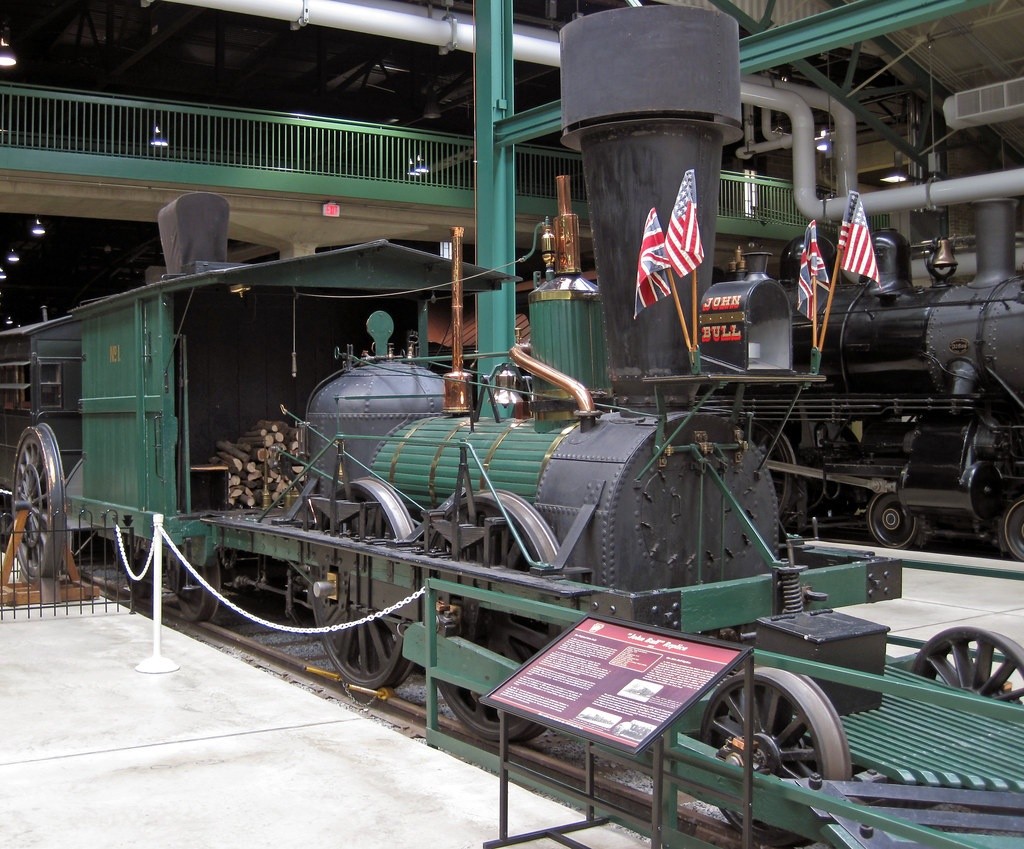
[[664, 169, 705, 278], [634, 205, 674, 319], [836, 190, 880, 289], [796, 217, 831, 319]]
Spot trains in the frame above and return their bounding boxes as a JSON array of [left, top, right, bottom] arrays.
[[0, 10, 1024, 849]]
